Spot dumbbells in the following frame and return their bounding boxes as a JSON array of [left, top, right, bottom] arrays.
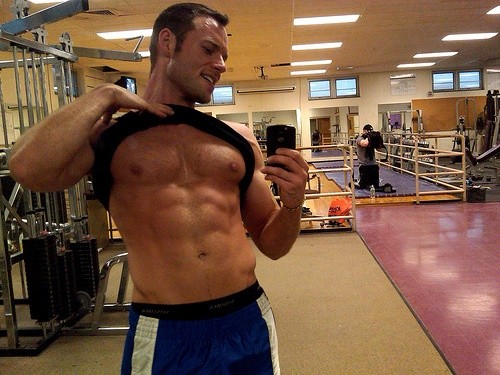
[[486, 174, 496, 182]]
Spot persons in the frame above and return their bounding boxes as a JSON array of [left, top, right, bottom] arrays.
[[312, 129, 319, 152], [9, 3, 310, 375], [349, 124, 382, 189]]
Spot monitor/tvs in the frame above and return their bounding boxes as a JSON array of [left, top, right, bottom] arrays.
[[115, 76, 137, 95]]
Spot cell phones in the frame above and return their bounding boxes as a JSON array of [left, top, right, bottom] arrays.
[[267, 125, 296, 173]]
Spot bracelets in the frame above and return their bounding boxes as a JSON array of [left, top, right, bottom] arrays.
[[281, 203, 306, 211]]
